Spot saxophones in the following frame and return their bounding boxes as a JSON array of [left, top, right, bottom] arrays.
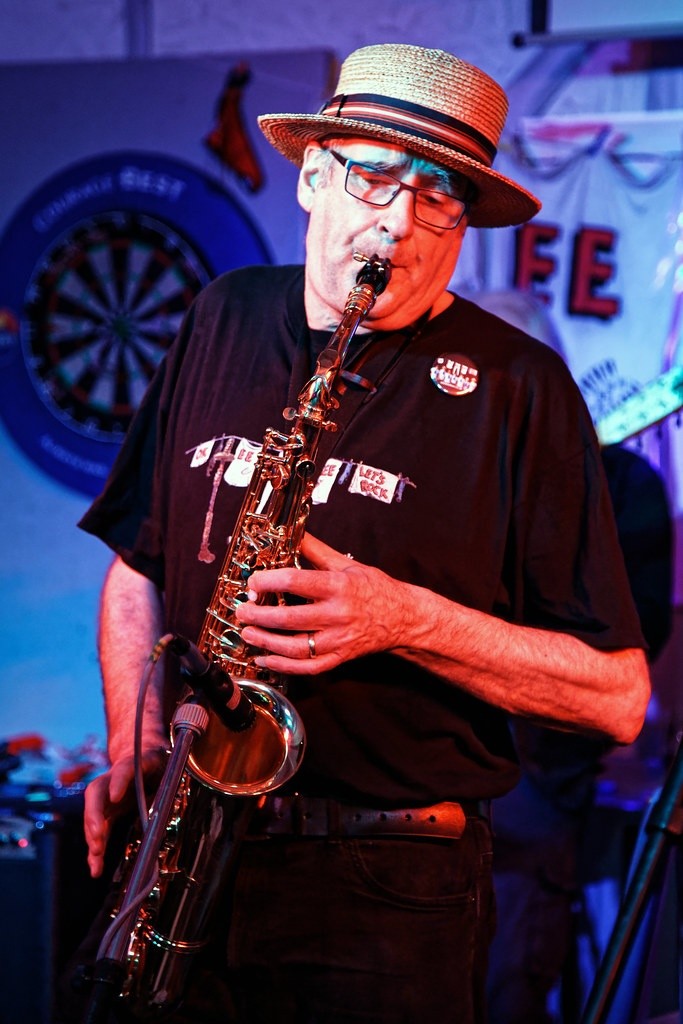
[[101, 250, 393, 1024]]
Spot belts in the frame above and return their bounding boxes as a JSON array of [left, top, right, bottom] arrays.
[[247, 797, 493, 840]]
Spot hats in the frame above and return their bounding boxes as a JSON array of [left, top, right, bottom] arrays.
[[259, 46, 542, 229]]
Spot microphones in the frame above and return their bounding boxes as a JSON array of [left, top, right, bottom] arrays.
[[168, 633, 257, 733]]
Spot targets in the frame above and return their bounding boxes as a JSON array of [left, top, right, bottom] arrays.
[[29, 218, 212, 434]]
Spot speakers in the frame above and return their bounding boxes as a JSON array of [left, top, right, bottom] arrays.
[[0, 817, 121, 1024]]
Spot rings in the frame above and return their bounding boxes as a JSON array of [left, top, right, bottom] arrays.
[[309, 631, 317, 660]]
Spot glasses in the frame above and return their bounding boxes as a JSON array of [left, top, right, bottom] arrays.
[[321, 144, 467, 230]]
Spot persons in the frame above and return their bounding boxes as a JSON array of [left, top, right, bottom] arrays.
[[74, 42, 652, 1024]]
